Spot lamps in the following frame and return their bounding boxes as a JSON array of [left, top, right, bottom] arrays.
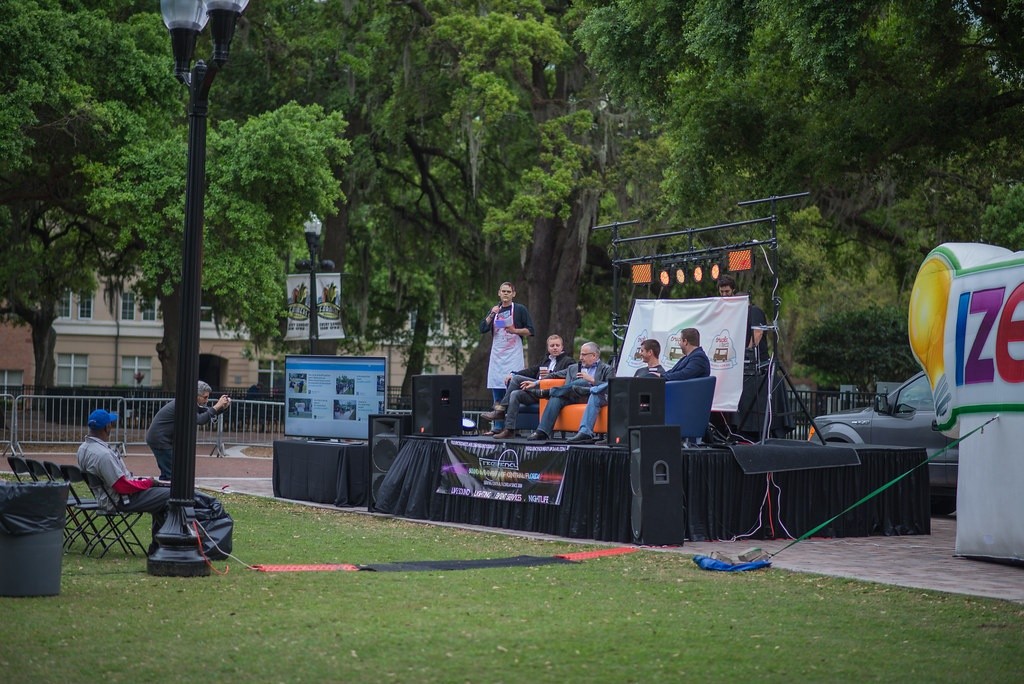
[[657, 260, 720, 286]]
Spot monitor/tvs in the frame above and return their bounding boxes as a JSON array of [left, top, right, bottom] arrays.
[[283, 354, 390, 445]]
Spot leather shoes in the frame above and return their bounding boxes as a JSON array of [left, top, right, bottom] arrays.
[[493, 429, 515, 438], [526, 431, 546, 441], [571, 385, 591, 399], [532, 388, 550, 400], [480, 409, 505, 421], [567, 432, 593, 445]]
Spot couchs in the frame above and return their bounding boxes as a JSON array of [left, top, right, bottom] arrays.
[[664, 378, 716, 446]]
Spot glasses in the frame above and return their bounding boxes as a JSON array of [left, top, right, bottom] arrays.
[[198, 394, 208, 399], [579, 352, 593, 356]]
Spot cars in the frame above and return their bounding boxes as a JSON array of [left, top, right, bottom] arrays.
[[807, 370, 959, 516]]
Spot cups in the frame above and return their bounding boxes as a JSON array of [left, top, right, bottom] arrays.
[[648, 368, 658, 376], [539, 367, 548, 375]]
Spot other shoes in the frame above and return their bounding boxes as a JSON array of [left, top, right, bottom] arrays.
[[596, 439, 607, 446], [484, 430, 494, 436]]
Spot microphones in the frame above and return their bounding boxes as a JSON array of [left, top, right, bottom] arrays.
[[490, 301, 502, 318], [578, 361, 582, 373]]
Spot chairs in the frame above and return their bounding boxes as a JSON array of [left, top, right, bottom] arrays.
[[7, 456, 148, 559], [514, 379, 607, 442]]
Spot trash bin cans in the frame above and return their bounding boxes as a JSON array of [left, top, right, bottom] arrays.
[[0, 481, 70, 596]]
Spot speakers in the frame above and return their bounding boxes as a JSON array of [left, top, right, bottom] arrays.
[[607, 377, 665, 448], [368, 414, 411, 513], [628, 425, 684, 546], [411, 374, 464, 437]]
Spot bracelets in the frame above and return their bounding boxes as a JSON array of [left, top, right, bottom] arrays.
[[490, 312, 495, 319], [534, 381, 538, 386]]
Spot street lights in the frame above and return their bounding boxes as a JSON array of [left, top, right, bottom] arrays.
[[304, 212, 325, 357], [141, 0, 251, 577]]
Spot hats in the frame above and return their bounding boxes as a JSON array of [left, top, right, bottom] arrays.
[[88, 409, 119, 430]]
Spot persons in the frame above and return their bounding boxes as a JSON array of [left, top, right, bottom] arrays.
[[648, 328, 710, 381], [480, 282, 535, 436], [146, 380, 231, 481], [289, 376, 305, 393], [734, 291, 770, 362], [519, 341, 616, 443], [77, 409, 170, 555], [245, 382, 263, 407], [481, 334, 577, 439], [567, 339, 665, 443], [717, 276, 737, 297]]
